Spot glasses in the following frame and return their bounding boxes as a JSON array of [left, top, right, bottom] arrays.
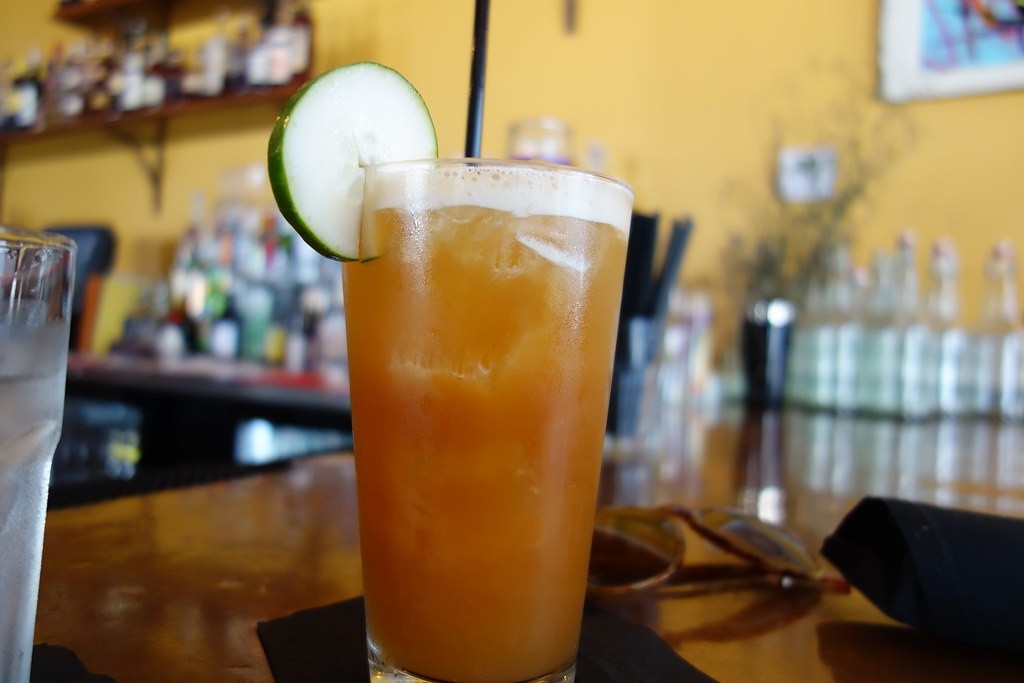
[[586, 504, 853, 597]]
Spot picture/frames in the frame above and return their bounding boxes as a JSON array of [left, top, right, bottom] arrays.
[[878, 1, 1024, 103]]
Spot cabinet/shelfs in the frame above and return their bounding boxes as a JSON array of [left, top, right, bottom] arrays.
[[1, 1, 304, 148]]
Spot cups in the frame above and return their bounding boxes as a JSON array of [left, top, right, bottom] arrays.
[[0, 223, 79, 683], [341, 160, 635, 683]]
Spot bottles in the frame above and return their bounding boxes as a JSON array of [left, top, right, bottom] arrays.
[[781, 232, 1024, 422]]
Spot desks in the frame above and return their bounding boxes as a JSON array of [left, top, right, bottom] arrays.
[[71, 356, 350, 491], [34, 398, 1023, 682]]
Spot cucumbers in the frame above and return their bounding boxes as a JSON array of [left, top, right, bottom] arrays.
[[267, 61, 439, 262]]
